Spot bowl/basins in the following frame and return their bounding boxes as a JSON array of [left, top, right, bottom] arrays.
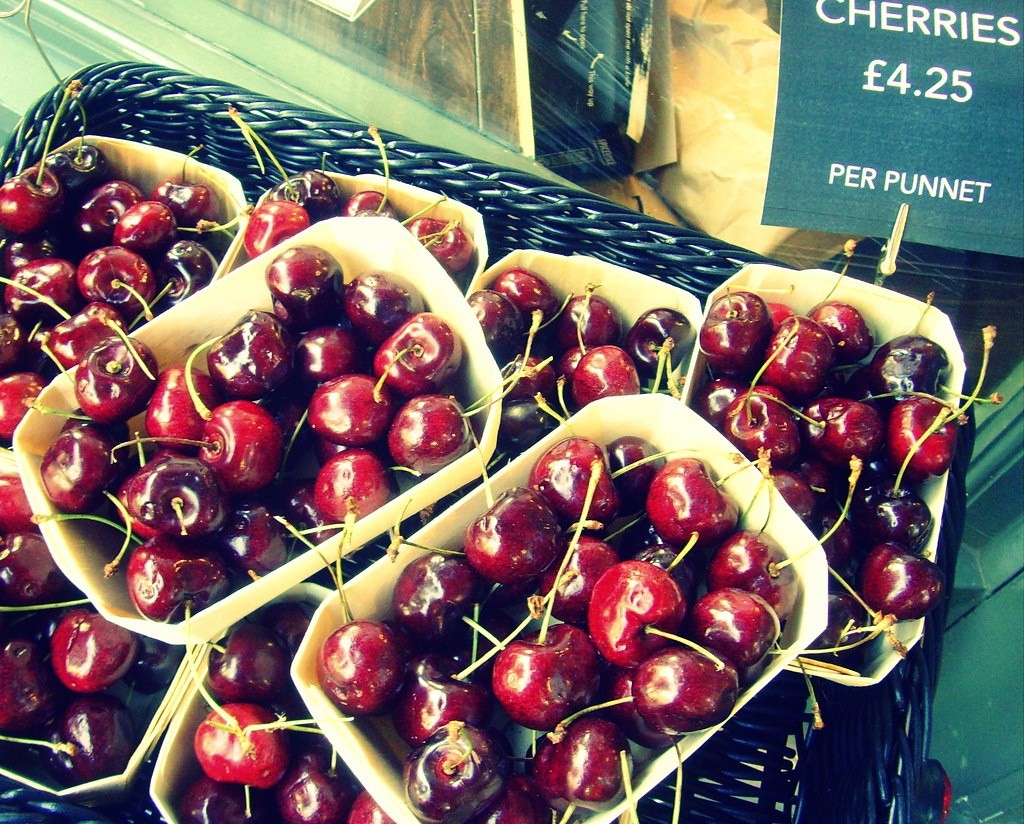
[[0, 132, 965, 824]]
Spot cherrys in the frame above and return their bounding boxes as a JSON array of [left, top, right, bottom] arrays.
[[0, 105, 958, 824]]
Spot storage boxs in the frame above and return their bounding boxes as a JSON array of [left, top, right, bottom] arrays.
[[0, 136, 966, 824], [523, 0, 677, 182]]
